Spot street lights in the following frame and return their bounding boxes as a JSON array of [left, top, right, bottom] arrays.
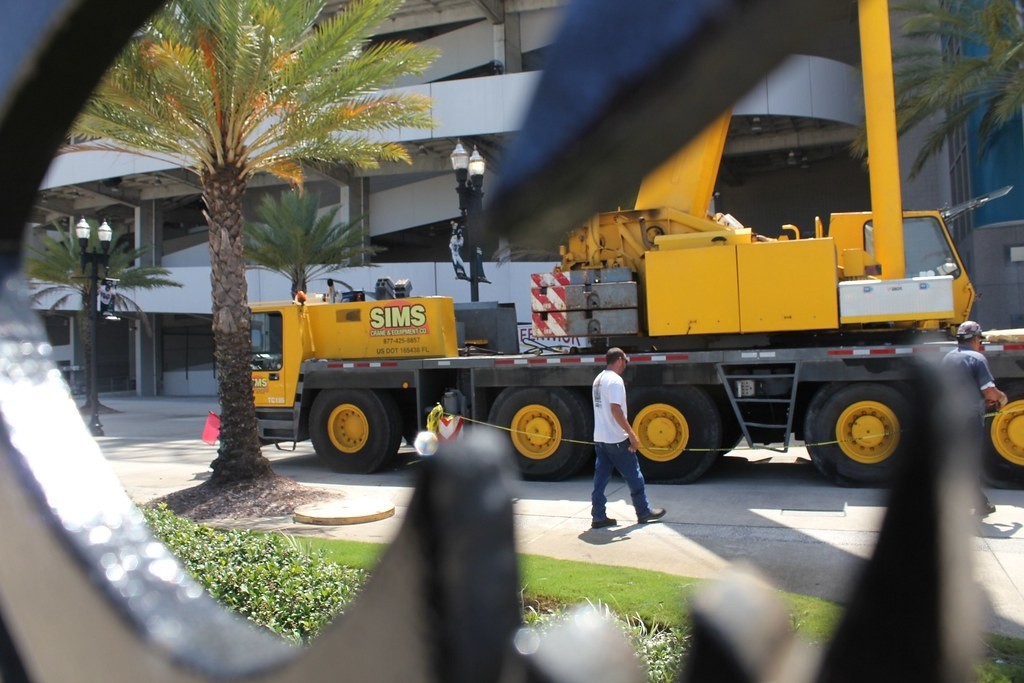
[[73, 213, 115, 439], [448, 140, 487, 303]]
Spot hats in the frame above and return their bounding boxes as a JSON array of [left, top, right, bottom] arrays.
[[956, 321, 986, 340]]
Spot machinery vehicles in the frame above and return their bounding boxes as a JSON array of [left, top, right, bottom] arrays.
[[248, 1, 1024, 491]]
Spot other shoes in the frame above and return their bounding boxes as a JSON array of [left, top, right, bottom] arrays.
[[973, 502, 995, 518], [592, 517, 617, 529], [638, 508, 665, 523]]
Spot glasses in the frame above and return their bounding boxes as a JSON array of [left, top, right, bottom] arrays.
[[617, 356, 628, 364]]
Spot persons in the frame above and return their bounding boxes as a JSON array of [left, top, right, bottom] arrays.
[[942, 321, 1008, 523], [592, 348, 666, 529]]
